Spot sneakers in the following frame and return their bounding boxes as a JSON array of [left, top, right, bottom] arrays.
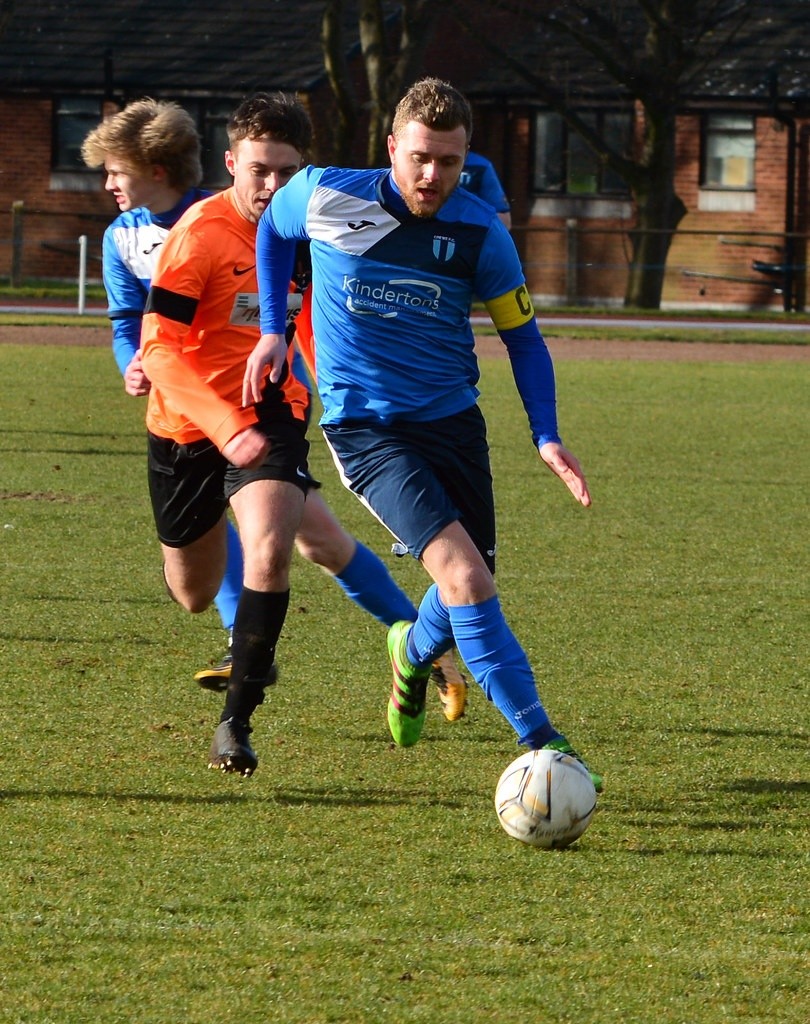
[[386, 619, 433, 749], [207, 715, 258, 778], [540, 738, 603, 794], [428, 647, 469, 722], [193, 655, 277, 693]]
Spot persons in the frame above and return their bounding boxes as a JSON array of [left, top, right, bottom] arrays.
[[241, 76, 600, 792], [79, 95, 466, 720], [459, 152, 512, 230], [139, 89, 311, 774]]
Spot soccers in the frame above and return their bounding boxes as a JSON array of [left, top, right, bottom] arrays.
[[494, 748, 597, 849]]
